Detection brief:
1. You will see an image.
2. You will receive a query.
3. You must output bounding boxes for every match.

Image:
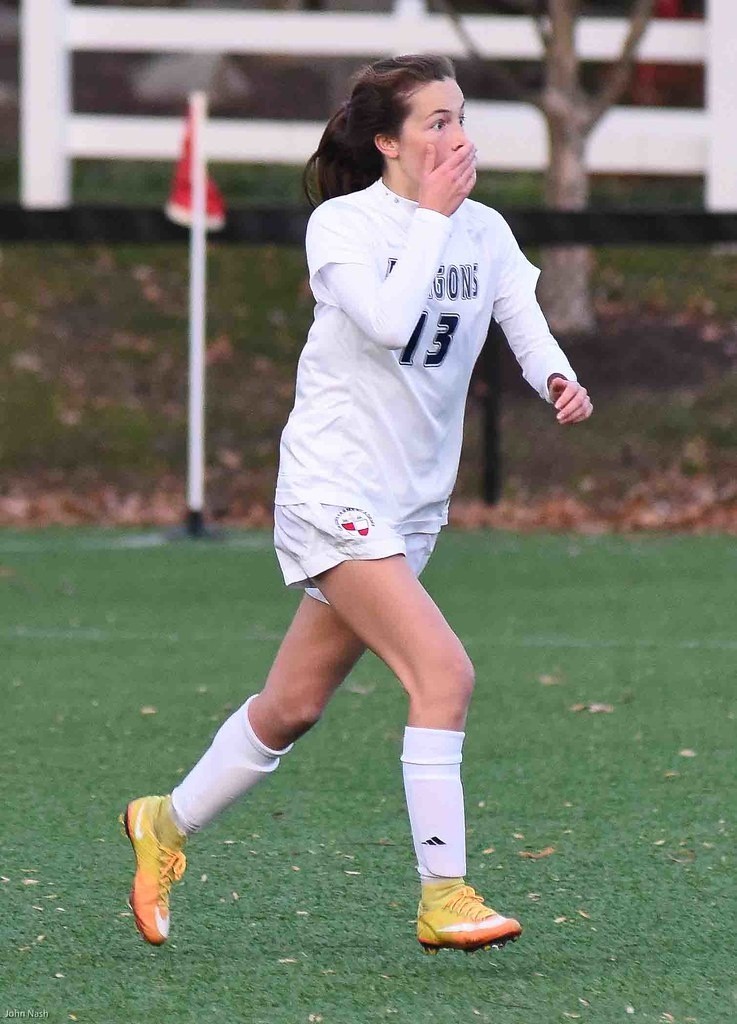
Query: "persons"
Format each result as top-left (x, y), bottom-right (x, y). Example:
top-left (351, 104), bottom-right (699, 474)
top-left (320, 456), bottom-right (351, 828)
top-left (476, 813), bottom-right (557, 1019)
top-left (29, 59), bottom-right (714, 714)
top-left (120, 52), bottom-right (597, 947)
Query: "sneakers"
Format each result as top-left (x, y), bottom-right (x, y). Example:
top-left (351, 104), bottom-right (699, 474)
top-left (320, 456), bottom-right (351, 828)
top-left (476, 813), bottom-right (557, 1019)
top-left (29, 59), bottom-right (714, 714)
top-left (416, 878), bottom-right (522, 957)
top-left (118, 796), bottom-right (186, 946)
